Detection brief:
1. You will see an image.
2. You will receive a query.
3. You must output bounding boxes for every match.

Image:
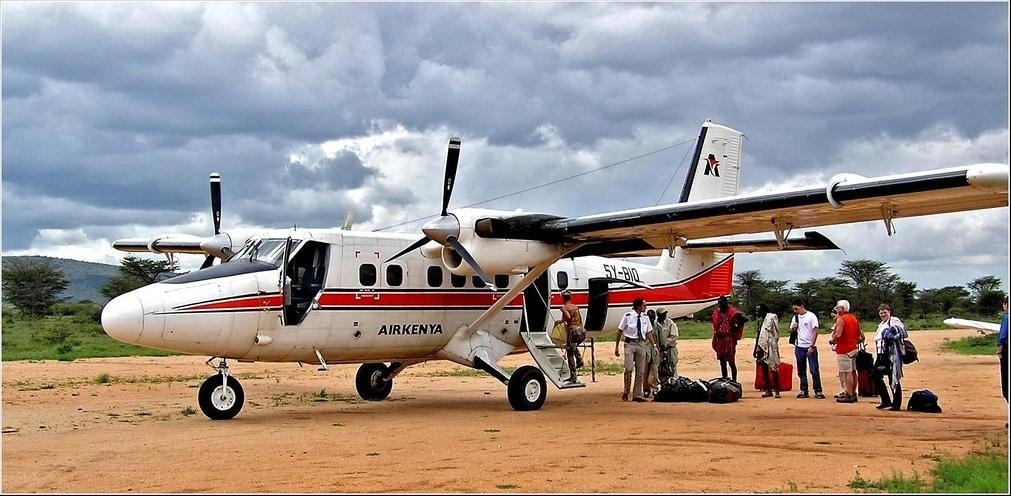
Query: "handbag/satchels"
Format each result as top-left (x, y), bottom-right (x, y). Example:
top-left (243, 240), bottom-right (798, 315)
top-left (901, 340), bottom-right (917, 364)
top-left (908, 390), bottom-right (942, 413)
top-left (657, 359), bottom-right (742, 403)
top-left (789, 329), bottom-right (798, 345)
top-left (857, 352), bottom-right (874, 369)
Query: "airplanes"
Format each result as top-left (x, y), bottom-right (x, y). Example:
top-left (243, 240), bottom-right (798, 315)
top-left (92, 121), bottom-right (1010, 423)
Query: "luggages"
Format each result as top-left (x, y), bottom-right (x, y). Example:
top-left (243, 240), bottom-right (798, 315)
top-left (857, 342), bottom-right (880, 397)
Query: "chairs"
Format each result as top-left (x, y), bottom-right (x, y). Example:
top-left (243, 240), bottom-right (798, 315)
top-left (288, 249), bottom-right (324, 309)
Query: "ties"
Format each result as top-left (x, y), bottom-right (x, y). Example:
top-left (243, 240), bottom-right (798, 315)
top-left (637, 315), bottom-right (644, 340)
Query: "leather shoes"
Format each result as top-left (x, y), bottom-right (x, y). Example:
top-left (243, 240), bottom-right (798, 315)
top-left (876, 402), bottom-right (890, 409)
top-left (887, 407), bottom-right (900, 411)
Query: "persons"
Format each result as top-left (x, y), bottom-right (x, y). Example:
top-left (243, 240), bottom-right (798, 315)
top-left (615, 297), bottom-right (657, 403)
top-left (829, 300), bottom-right (861, 403)
top-left (555, 289), bottom-right (586, 384)
top-left (831, 310), bottom-right (847, 399)
top-left (789, 296), bottom-right (826, 399)
top-left (652, 306), bottom-right (679, 380)
top-left (995, 296), bottom-right (1008, 427)
top-left (712, 296), bottom-right (749, 384)
top-left (753, 305), bottom-right (781, 398)
top-left (872, 304), bottom-right (906, 411)
top-left (643, 309), bottom-right (659, 398)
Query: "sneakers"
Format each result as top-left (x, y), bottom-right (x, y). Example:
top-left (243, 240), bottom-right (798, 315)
top-left (814, 391), bottom-right (825, 399)
top-left (622, 390), bottom-right (658, 402)
top-left (575, 362), bottom-right (583, 368)
top-left (835, 390), bottom-right (857, 403)
top-left (798, 391), bottom-right (809, 398)
top-left (762, 392), bottom-right (781, 398)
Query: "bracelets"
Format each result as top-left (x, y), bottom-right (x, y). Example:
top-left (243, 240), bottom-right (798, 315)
top-left (653, 344), bottom-right (657, 347)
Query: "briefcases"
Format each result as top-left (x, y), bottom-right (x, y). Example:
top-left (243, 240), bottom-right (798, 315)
top-left (755, 361), bottom-right (793, 391)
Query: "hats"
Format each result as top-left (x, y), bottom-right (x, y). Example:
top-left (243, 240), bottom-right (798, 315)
top-left (656, 308), bottom-right (668, 316)
